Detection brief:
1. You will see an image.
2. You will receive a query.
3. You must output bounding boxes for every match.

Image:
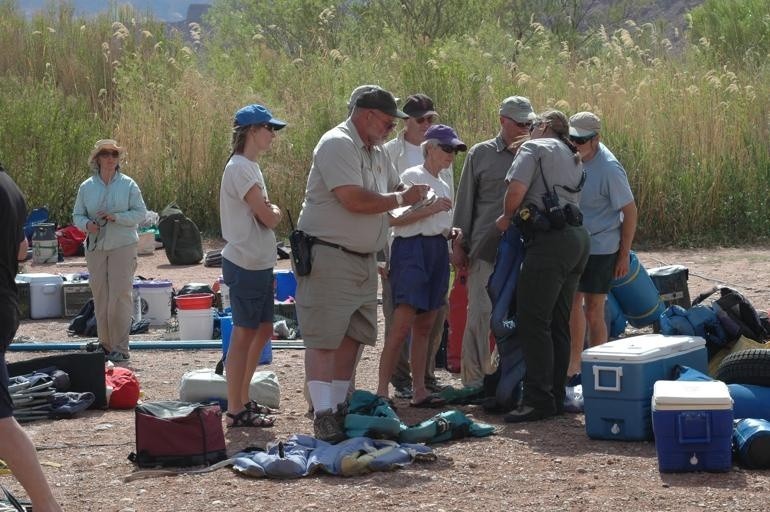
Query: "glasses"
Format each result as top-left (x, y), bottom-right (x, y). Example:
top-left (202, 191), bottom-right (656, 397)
top-left (99, 151), bottom-right (119, 157)
top-left (569, 135), bottom-right (593, 145)
top-left (412, 116), bottom-right (434, 123)
top-left (438, 143), bottom-right (459, 155)
top-left (508, 117), bottom-right (531, 127)
top-left (373, 114), bottom-right (397, 129)
top-left (260, 123), bottom-right (274, 131)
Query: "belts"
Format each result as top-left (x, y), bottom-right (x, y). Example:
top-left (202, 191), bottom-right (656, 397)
top-left (313, 238), bottom-right (368, 258)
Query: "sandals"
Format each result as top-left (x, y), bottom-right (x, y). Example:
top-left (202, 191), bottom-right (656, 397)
top-left (244, 399), bottom-right (277, 414)
top-left (226, 410), bottom-right (276, 427)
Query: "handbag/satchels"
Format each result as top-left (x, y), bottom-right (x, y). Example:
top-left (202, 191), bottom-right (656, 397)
top-left (105, 360), bottom-right (139, 408)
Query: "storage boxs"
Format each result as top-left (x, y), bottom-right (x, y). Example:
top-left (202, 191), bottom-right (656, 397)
top-left (581, 334), bottom-right (708, 443)
top-left (651, 379), bottom-right (732, 474)
top-left (16, 273), bottom-right (63, 318)
top-left (132, 281), bottom-right (172, 326)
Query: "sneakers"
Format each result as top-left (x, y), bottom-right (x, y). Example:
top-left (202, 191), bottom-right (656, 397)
top-left (424, 378), bottom-right (450, 392)
top-left (105, 351), bottom-right (130, 361)
top-left (333, 401), bottom-right (348, 427)
top-left (394, 386), bottom-right (414, 399)
top-left (503, 402), bottom-right (556, 422)
top-left (93, 345), bottom-right (108, 354)
top-left (314, 408), bottom-right (342, 445)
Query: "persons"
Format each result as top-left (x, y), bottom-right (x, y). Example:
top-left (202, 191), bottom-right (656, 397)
top-left (496, 109), bottom-right (592, 423)
top-left (219, 104), bottom-right (285, 427)
top-left (289, 91), bottom-right (410, 446)
top-left (304, 84), bottom-right (401, 415)
top-left (1, 169), bottom-right (63, 511)
top-left (558, 112), bottom-right (638, 387)
top-left (73, 140), bottom-right (146, 362)
top-left (376, 122), bottom-right (468, 408)
top-left (384, 93), bottom-right (456, 400)
top-left (452, 96), bottom-right (541, 392)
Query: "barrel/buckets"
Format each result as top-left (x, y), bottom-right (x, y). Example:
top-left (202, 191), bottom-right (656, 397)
top-left (176, 308), bottom-right (214, 340)
top-left (30, 222), bottom-right (59, 264)
top-left (139, 281), bottom-right (173, 321)
top-left (218, 305), bottom-right (273, 365)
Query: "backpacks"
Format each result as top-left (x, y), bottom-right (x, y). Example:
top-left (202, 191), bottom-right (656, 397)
top-left (158, 203), bottom-right (203, 265)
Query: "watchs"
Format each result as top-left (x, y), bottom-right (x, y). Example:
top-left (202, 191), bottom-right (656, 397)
top-left (394, 192), bottom-right (404, 208)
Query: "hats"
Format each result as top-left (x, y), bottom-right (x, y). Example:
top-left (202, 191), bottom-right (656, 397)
top-left (424, 124), bottom-right (467, 152)
top-left (568, 111), bottom-right (601, 137)
top-left (355, 89), bottom-right (410, 119)
top-left (499, 95), bottom-right (537, 123)
top-left (88, 139), bottom-right (123, 169)
top-left (235, 104), bottom-right (287, 131)
top-left (402, 93), bottom-right (440, 119)
top-left (347, 85), bottom-right (401, 110)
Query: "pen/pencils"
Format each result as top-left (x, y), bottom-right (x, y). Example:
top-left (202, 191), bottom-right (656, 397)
top-left (407, 180), bottom-right (417, 186)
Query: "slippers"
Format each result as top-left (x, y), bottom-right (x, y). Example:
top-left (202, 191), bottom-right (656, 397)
top-left (375, 395), bottom-right (397, 412)
top-left (409, 395), bottom-right (447, 408)
